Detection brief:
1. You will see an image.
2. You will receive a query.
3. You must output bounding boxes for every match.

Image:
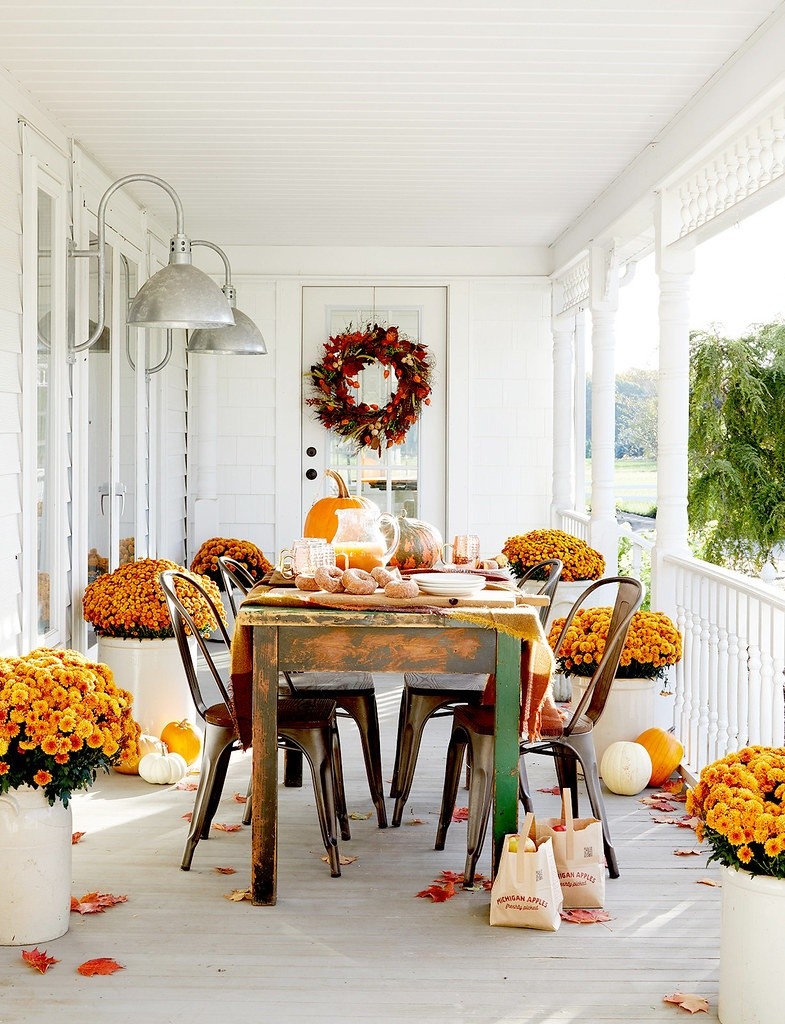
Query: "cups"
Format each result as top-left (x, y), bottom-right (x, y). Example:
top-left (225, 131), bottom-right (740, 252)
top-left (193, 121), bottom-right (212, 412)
top-left (439, 533), bottom-right (480, 566)
top-left (277, 537), bottom-right (349, 580)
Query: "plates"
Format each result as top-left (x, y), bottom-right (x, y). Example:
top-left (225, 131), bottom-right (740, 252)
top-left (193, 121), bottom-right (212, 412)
top-left (411, 572), bottom-right (486, 598)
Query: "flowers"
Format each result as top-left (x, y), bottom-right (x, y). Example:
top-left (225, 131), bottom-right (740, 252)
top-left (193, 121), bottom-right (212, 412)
top-left (0, 647), bottom-right (141, 810)
top-left (33, 535), bottom-right (135, 634)
top-left (81, 556), bottom-right (229, 639)
top-left (545, 604), bottom-right (683, 696)
top-left (302, 315), bottom-right (437, 459)
top-left (190, 537), bottom-right (276, 592)
top-left (686, 743), bottom-right (785, 881)
top-left (501, 528), bottom-right (607, 581)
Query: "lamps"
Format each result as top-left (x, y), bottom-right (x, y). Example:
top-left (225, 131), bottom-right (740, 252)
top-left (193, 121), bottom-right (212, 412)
top-left (66, 174), bottom-right (238, 353)
top-left (118, 239), bottom-right (268, 355)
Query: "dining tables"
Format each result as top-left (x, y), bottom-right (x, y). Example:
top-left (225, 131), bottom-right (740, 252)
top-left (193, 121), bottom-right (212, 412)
top-left (236, 567), bottom-right (542, 909)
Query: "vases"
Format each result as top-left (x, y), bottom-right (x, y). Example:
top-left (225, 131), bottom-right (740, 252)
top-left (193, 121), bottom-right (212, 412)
top-left (516, 577), bottom-right (603, 637)
top-left (198, 587), bottom-right (254, 643)
top-left (0, 778), bottom-right (74, 946)
top-left (569, 673), bottom-right (677, 780)
top-left (717, 862), bottom-right (785, 1023)
top-left (97, 635), bottom-right (198, 771)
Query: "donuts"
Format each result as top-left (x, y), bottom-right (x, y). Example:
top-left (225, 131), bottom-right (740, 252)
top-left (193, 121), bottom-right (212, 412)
top-left (296, 566), bottom-right (418, 599)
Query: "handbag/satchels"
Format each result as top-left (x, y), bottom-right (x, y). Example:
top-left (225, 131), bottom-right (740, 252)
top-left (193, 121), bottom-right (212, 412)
top-left (546, 788), bottom-right (605, 910)
top-left (490, 812), bottom-right (564, 933)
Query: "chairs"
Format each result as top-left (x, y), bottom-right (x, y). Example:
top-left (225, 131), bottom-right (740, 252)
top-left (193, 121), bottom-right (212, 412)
top-left (435, 576), bottom-right (646, 890)
top-left (219, 555), bottom-right (388, 831)
top-left (159, 570), bottom-right (351, 879)
top-left (390, 557), bottom-right (563, 824)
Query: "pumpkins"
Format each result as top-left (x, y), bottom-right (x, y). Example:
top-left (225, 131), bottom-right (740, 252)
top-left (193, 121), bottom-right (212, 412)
top-left (600, 728), bottom-right (684, 796)
top-left (304, 468), bottom-right (443, 571)
top-left (113, 720), bottom-right (205, 785)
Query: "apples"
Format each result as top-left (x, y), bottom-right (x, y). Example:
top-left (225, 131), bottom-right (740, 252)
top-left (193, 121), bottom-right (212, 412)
top-left (508, 825), bottom-right (567, 853)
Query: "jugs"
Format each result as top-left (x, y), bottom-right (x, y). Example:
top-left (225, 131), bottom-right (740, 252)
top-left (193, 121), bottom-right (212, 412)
top-left (331, 508), bottom-right (399, 573)
